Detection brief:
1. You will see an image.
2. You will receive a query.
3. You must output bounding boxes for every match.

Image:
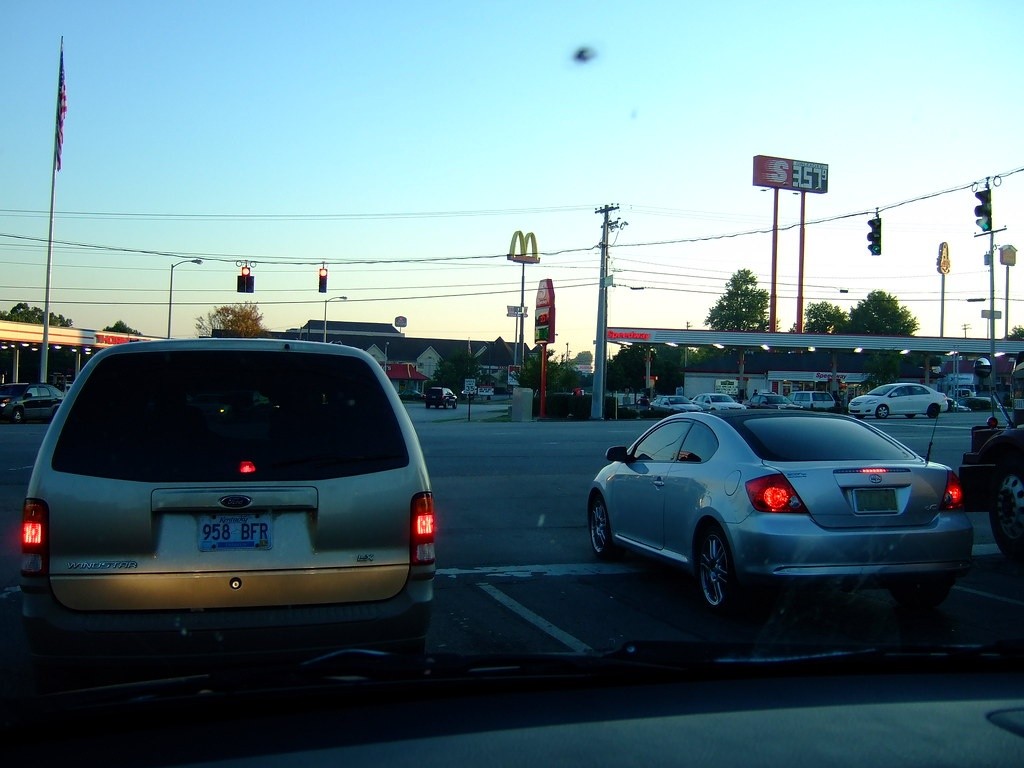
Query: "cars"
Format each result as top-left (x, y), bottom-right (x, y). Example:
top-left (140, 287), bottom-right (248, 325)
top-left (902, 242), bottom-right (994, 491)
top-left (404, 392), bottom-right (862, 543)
top-left (585, 409), bottom-right (975, 615)
top-left (848, 382), bottom-right (949, 419)
top-left (742, 393), bottom-right (804, 410)
top-left (0, 383), bottom-right (66, 423)
top-left (689, 393), bottom-right (747, 410)
top-left (649, 395), bottom-right (704, 411)
top-left (397, 389), bottom-right (426, 400)
top-left (787, 392), bottom-right (836, 412)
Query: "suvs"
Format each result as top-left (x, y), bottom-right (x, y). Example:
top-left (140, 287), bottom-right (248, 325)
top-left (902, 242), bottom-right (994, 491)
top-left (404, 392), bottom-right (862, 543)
top-left (16, 337), bottom-right (438, 666)
top-left (426, 386), bottom-right (458, 409)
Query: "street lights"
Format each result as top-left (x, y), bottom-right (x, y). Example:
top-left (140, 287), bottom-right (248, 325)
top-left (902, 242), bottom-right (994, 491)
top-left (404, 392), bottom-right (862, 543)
top-left (384, 341), bottom-right (389, 373)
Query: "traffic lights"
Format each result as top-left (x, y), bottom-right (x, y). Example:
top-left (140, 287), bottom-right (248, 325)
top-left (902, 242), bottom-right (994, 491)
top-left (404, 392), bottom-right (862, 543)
top-left (975, 190), bottom-right (992, 231)
top-left (319, 269), bottom-right (327, 293)
top-left (867, 218), bottom-right (881, 255)
top-left (238, 267), bottom-right (254, 292)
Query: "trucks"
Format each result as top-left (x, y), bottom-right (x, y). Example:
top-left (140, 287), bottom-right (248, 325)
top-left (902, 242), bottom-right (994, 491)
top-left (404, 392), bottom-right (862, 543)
top-left (958, 351), bottom-right (1024, 560)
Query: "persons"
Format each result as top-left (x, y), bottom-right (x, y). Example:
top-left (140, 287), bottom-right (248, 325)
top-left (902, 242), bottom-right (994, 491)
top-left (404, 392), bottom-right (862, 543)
top-left (636, 396), bottom-right (650, 420)
top-left (753, 389), bottom-right (758, 396)
top-left (573, 386), bottom-right (580, 396)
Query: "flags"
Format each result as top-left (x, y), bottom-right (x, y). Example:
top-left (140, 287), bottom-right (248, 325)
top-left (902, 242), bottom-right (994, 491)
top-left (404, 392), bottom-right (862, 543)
top-left (57, 35), bottom-right (68, 174)
